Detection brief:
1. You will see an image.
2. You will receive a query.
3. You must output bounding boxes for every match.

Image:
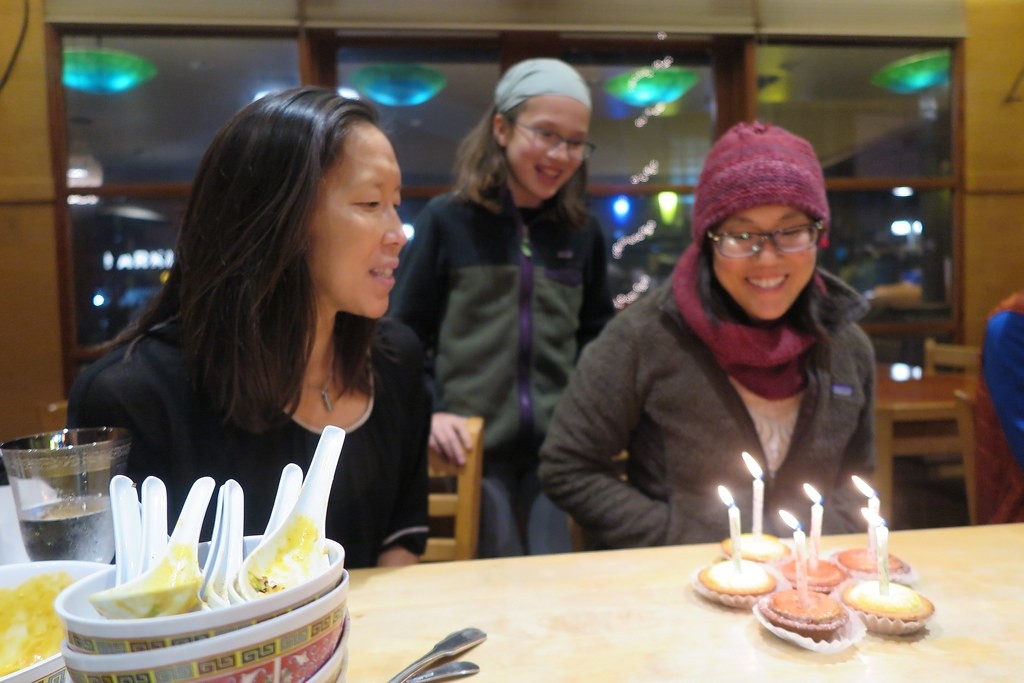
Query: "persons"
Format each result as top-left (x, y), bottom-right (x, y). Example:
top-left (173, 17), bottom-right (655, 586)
top-left (66, 82), bottom-right (434, 571)
top-left (382, 58), bottom-right (618, 559)
top-left (541, 117), bottom-right (877, 552)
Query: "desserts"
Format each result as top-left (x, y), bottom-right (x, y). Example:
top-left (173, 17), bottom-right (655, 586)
top-left (757, 592), bottom-right (849, 641)
top-left (841, 581), bottom-right (934, 623)
top-left (782, 558), bottom-right (849, 593)
top-left (722, 533), bottom-right (791, 564)
top-left (830, 549), bottom-right (910, 576)
top-left (697, 562), bottom-right (776, 597)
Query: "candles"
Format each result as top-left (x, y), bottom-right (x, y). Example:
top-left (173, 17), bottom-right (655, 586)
top-left (719, 452), bottom-right (890, 605)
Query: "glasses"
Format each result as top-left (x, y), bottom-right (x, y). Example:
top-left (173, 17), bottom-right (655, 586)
top-left (516, 120), bottom-right (595, 160)
top-left (707, 218), bottom-right (823, 257)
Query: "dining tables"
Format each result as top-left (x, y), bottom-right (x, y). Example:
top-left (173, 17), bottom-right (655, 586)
top-left (340, 522), bottom-right (1024, 683)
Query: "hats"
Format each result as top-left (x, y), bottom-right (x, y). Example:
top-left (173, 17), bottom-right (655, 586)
top-left (692, 122), bottom-right (828, 242)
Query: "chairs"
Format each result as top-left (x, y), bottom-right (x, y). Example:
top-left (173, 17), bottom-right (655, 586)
top-left (924, 336), bottom-right (980, 380)
top-left (873, 402), bottom-right (976, 526)
top-left (419, 416), bottom-right (484, 562)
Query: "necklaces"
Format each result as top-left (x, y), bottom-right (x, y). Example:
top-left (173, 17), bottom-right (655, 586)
top-left (303, 355), bottom-right (335, 412)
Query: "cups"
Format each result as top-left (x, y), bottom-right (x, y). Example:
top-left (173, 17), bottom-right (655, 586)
top-left (0, 425), bottom-right (131, 566)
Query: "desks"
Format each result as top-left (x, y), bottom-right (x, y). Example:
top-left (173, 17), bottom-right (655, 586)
top-left (878, 363), bottom-right (973, 401)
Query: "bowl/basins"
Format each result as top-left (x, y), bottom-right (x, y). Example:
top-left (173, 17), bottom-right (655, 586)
top-left (0, 559), bottom-right (112, 683)
top-left (66, 609), bottom-right (350, 683)
top-left (336, 649), bottom-right (348, 683)
top-left (62, 570), bottom-right (349, 683)
top-left (55, 537), bottom-right (345, 648)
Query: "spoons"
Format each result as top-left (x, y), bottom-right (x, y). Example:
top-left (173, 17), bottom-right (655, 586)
top-left (87, 425), bottom-right (346, 618)
